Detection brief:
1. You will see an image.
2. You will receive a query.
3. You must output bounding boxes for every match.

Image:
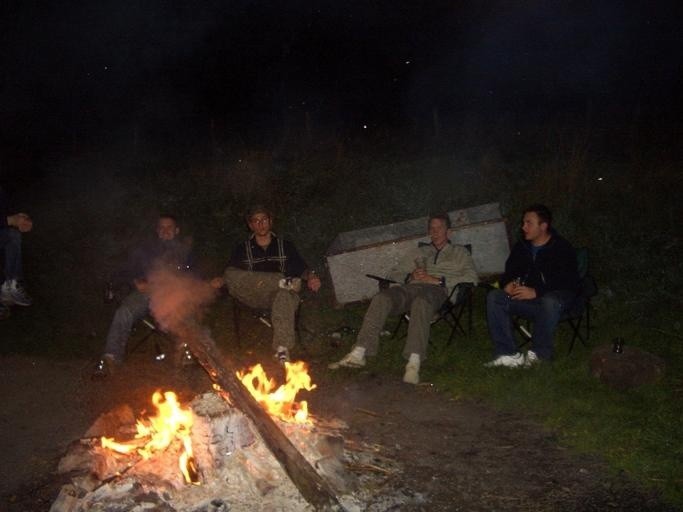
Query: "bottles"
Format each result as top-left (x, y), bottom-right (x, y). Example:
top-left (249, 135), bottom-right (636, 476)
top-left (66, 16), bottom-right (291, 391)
top-left (612, 335), bottom-right (625, 368)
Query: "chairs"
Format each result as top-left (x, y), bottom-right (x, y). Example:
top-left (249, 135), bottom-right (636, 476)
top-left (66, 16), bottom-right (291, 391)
top-left (222, 255), bottom-right (323, 354)
top-left (366, 240), bottom-right (475, 351)
top-left (101, 244), bottom-right (222, 361)
top-left (478, 245), bottom-right (595, 359)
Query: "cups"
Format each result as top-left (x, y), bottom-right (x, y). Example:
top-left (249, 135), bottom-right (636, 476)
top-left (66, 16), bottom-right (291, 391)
top-left (512, 273), bottom-right (528, 290)
top-left (415, 255), bottom-right (427, 273)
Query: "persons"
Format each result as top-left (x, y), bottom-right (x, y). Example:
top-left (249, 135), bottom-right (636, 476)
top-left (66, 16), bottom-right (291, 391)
top-left (479, 202), bottom-right (588, 371)
top-left (207, 203), bottom-right (321, 369)
top-left (326, 209), bottom-right (480, 385)
top-left (0, 212), bottom-right (35, 308)
top-left (89, 214), bottom-right (206, 374)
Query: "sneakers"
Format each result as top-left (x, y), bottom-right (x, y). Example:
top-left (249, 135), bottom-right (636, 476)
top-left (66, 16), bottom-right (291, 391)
top-left (483, 352), bottom-right (526, 370)
top-left (90, 358), bottom-right (109, 380)
top-left (1, 278), bottom-right (34, 307)
top-left (402, 361), bottom-right (420, 386)
top-left (326, 351), bottom-right (368, 370)
top-left (279, 275), bottom-right (302, 292)
top-left (523, 350), bottom-right (539, 371)
top-left (274, 346), bottom-right (289, 363)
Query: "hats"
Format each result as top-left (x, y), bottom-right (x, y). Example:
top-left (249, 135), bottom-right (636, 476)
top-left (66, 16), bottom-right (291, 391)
top-left (245, 205), bottom-right (275, 224)
